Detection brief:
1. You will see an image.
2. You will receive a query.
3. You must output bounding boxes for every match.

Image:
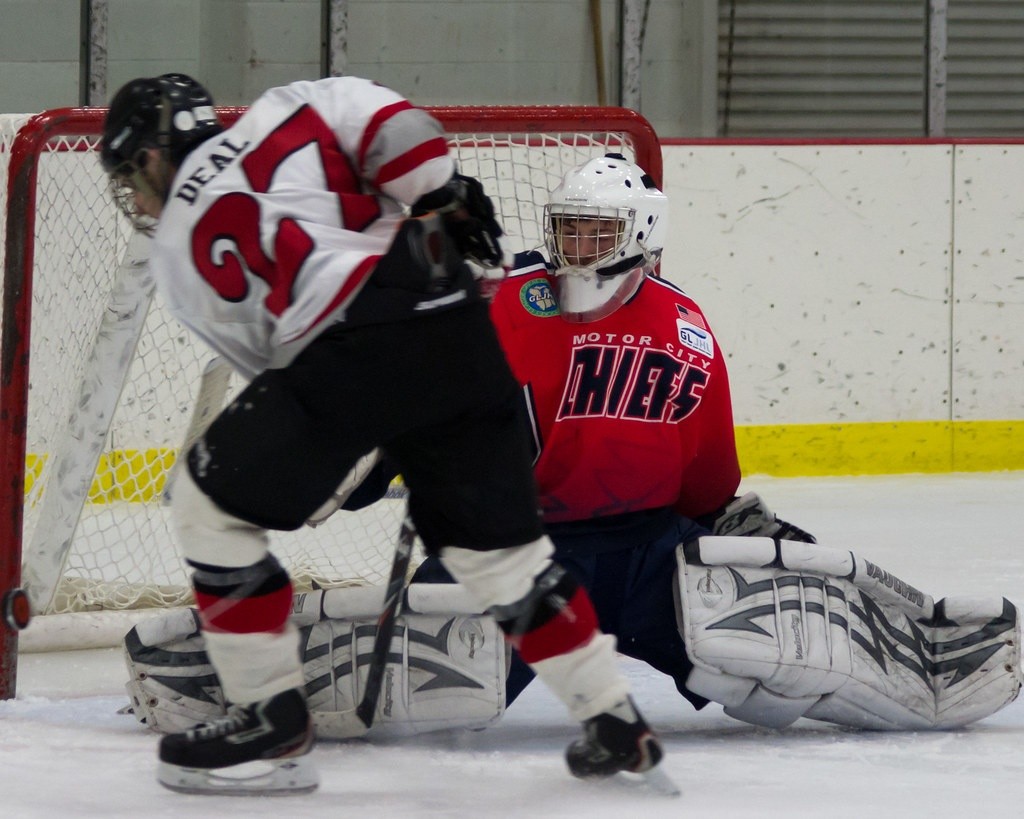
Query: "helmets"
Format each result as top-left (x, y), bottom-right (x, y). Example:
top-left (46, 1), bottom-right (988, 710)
top-left (542, 153), bottom-right (668, 323)
top-left (100, 73), bottom-right (229, 239)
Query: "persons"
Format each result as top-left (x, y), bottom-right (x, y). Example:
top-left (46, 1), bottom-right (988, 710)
top-left (332, 146), bottom-right (1022, 730)
top-left (102, 71), bottom-right (665, 779)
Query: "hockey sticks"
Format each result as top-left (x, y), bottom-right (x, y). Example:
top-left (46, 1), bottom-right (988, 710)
top-left (308, 483), bottom-right (426, 742)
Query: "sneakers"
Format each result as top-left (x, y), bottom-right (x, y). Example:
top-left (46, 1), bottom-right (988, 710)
top-left (564, 692), bottom-right (679, 798)
top-left (157, 686), bottom-right (320, 798)
